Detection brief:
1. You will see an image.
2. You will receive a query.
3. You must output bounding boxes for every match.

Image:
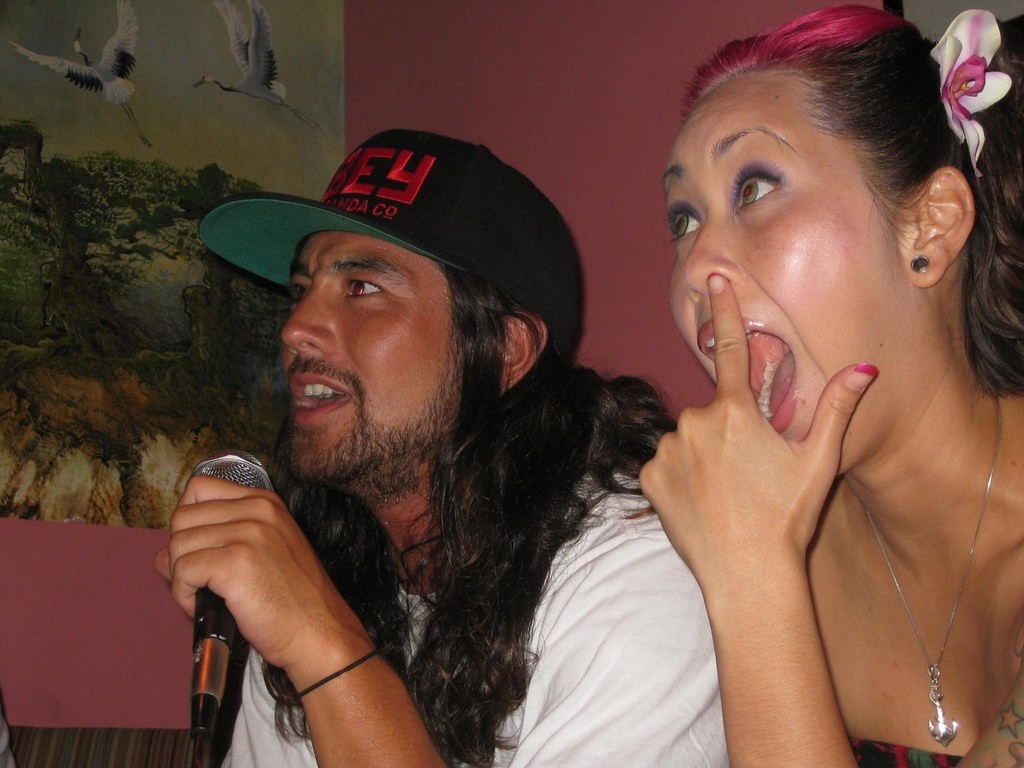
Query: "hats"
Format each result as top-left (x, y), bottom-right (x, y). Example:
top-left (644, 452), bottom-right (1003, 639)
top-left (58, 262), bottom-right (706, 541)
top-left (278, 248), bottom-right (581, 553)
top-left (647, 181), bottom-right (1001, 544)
top-left (195, 129), bottom-right (588, 356)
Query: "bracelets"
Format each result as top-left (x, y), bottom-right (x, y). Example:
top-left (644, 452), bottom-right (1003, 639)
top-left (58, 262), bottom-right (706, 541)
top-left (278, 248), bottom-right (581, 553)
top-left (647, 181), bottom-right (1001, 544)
top-left (296, 650), bottom-right (378, 697)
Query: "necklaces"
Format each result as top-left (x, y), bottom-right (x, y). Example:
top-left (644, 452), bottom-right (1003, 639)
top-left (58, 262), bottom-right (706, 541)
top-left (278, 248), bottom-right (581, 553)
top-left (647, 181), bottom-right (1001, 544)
top-left (861, 395), bottom-right (1005, 749)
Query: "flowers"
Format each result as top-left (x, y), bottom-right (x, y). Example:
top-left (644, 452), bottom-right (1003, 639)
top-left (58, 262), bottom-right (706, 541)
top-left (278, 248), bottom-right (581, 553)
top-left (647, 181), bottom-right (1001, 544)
top-left (928, 9), bottom-right (1014, 179)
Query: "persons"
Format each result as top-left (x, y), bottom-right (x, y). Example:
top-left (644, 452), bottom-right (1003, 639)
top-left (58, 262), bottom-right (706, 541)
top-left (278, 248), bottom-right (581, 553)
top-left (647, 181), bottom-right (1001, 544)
top-left (633, 0), bottom-right (1024, 767)
top-left (148, 125), bottom-right (733, 768)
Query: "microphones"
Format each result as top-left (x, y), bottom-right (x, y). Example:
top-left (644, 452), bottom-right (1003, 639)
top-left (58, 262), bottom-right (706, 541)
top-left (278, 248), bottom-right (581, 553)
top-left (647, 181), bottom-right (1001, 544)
top-left (180, 446), bottom-right (279, 747)
